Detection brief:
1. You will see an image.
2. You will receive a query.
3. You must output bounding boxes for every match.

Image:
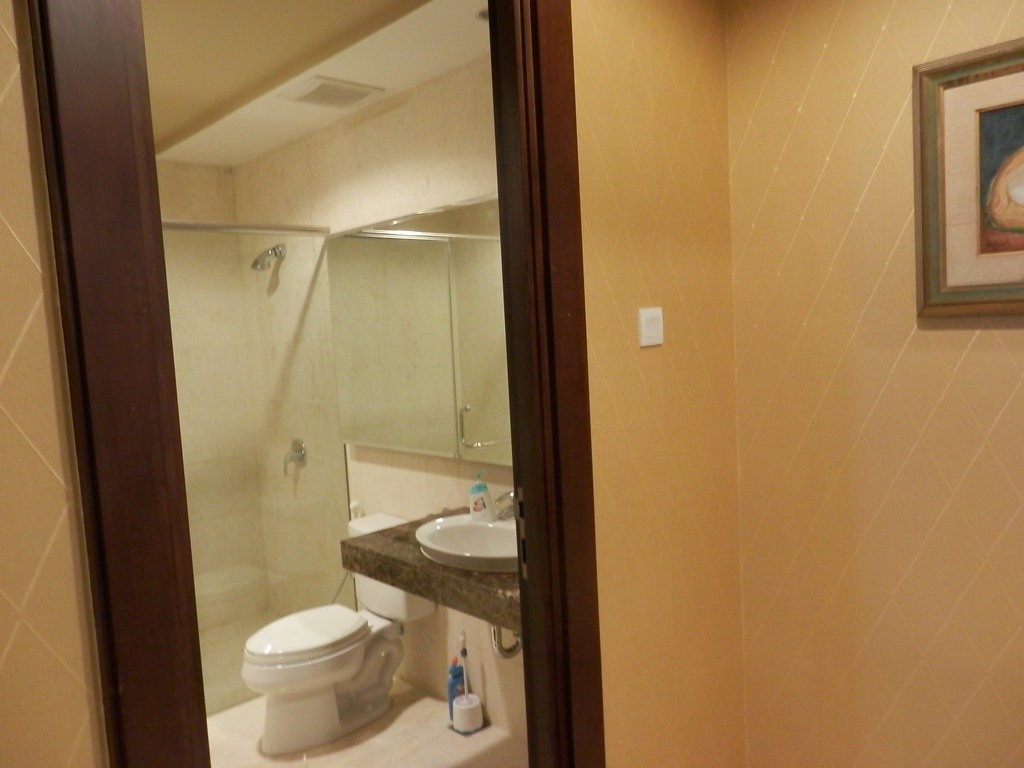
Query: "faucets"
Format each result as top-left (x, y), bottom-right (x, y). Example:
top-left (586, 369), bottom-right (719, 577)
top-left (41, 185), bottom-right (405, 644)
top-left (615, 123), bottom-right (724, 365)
top-left (490, 486), bottom-right (517, 523)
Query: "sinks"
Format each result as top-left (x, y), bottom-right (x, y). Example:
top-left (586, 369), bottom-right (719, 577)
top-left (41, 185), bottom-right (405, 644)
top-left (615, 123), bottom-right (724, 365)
top-left (413, 509), bottom-right (521, 576)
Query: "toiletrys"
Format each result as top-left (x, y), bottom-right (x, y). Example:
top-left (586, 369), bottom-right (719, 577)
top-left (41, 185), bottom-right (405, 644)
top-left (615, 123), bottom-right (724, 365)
top-left (468, 467), bottom-right (492, 519)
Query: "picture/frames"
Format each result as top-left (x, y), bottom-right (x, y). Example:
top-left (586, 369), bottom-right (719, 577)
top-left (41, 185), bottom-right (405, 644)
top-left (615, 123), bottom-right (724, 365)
top-left (912, 34), bottom-right (1023, 321)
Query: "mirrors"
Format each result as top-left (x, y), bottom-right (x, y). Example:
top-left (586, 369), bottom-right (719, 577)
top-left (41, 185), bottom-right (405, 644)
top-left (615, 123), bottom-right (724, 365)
top-left (324, 191), bottom-right (513, 468)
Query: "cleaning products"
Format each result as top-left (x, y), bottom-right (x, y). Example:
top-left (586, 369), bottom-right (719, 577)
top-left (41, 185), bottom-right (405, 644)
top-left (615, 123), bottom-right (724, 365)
top-left (445, 657), bottom-right (472, 724)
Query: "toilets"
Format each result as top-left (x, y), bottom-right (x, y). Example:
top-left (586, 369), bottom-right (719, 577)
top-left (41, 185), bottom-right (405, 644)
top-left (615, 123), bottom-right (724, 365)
top-left (237, 508), bottom-right (437, 760)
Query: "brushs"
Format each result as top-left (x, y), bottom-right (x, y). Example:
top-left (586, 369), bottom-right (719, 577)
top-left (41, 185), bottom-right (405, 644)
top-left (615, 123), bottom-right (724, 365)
top-left (452, 628), bottom-right (485, 735)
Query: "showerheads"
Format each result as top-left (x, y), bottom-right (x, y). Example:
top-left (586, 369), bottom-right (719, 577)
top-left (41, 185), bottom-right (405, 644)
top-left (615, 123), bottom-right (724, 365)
top-left (248, 242), bottom-right (288, 272)
